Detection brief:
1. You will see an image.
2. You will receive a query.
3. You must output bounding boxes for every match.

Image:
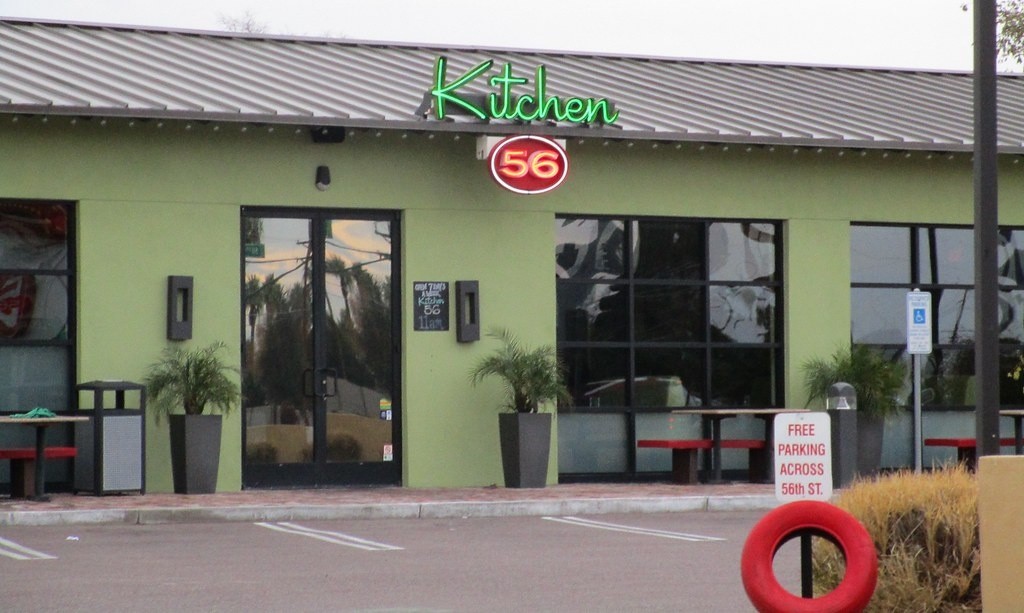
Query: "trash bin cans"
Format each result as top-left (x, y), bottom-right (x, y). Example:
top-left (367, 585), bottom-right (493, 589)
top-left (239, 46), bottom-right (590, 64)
top-left (72, 379), bottom-right (149, 497)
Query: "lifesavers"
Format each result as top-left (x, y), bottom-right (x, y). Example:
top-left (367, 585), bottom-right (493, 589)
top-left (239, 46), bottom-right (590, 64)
top-left (742, 498), bottom-right (877, 613)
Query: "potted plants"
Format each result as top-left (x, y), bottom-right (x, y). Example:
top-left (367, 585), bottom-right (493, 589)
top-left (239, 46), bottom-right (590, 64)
top-left (464, 324), bottom-right (574, 489)
top-left (800, 340), bottom-right (909, 481)
top-left (139, 340), bottom-right (248, 494)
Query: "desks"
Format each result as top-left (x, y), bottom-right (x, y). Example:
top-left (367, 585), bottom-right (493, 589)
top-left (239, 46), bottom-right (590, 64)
top-left (973, 410), bottom-right (1024, 456)
top-left (0, 415), bottom-right (90, 503)
top-left (672, 409), bottom-right (811, 486)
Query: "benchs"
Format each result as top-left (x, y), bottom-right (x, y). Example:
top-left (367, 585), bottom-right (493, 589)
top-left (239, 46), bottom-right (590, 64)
top-left (636, 439), bottom-right (774, 485)
top-left (0, 447), bottom-right (79, 500)
top-left (924, 437), bottom-right (1024, 475)
